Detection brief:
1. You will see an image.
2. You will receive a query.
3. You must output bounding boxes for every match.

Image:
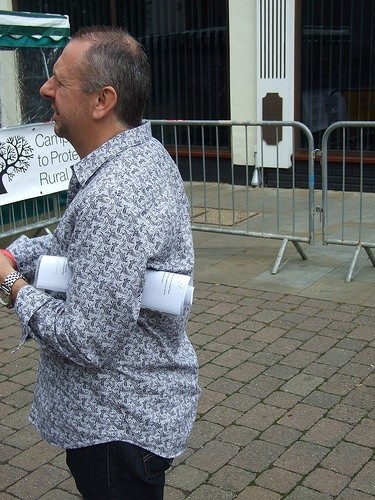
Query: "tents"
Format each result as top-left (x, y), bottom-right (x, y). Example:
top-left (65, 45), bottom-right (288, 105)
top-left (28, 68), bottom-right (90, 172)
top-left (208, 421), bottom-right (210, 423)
top-left (0, 9), bottom-right (71, 80)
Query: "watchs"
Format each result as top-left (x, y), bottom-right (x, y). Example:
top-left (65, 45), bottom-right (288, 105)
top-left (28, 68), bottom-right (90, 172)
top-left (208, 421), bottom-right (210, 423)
top-left (0, 271), bottom-right (26, 309)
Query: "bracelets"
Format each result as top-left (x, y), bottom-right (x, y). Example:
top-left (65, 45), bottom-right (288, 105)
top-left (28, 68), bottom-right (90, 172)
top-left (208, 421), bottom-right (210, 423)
top-left (0, 248), bottom-right (17, 270)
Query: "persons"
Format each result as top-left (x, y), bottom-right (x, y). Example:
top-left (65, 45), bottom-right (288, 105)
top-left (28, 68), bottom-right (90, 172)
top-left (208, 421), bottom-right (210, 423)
top-left (0, 25), bottom-right (201, 500)
